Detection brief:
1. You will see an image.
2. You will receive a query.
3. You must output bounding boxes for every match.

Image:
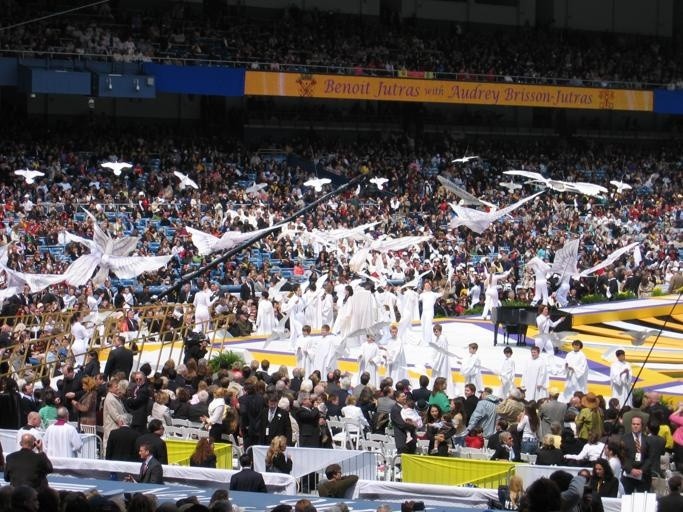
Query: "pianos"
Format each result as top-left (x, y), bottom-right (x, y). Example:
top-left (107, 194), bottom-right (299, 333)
top-left (494, 305), bottom-right (572, 346)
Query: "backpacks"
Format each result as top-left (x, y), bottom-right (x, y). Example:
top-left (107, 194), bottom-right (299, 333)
top-left (371, 412), bottom-right (389, 434)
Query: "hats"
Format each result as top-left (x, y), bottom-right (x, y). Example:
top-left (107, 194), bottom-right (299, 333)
top-left (511, 388), bottom-right (520, 398)
top-left (549, 387), bottom-right (559, 397)
top-left (517, 385), bottom-right (526, 391)
top-left (477, 387), bottom-right (492, 393)
top-left (416, 400), bottom-right (428, 411)
top-left (581, 392), bottom-right (600, 408)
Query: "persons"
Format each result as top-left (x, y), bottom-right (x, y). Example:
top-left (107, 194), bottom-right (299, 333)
top-left (0, 2), bottom-right (682, 511)
